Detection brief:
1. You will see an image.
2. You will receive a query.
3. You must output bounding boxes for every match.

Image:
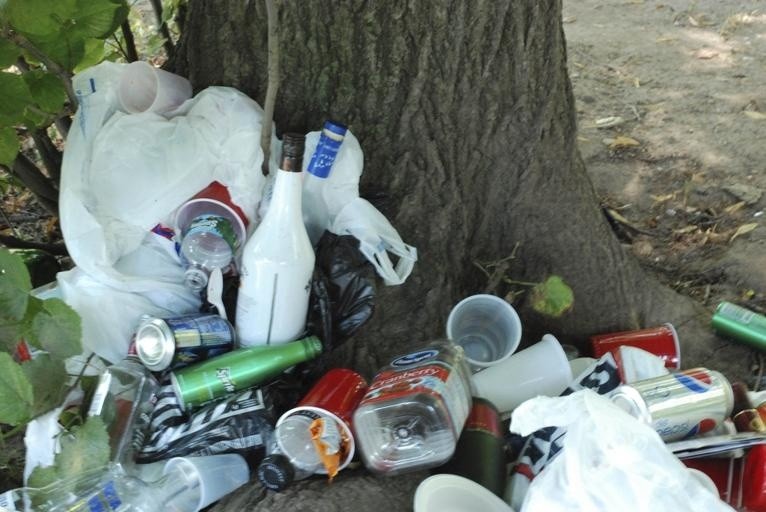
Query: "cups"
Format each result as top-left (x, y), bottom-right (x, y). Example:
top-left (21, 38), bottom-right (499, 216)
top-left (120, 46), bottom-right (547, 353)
top-left (115, 60), bottom-right (193, 116)
top-left (175, 181), bottom-right (248, 252)
top-left (470, 333), bottom-right (574, 416)
top-left (274, 370), bottom-right (368, 476)
top-left (161, 453), bottom-right (249, 512)
top-left (446, 292), bottom-right (521, 371)
top-left (592, 322), bottom-right (682, 383)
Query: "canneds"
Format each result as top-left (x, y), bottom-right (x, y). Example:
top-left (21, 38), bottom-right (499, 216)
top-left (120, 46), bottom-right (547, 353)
top-left (610, 364), bottom-right (735, 443)
top-left (135, 314), bottom-right (234, 374)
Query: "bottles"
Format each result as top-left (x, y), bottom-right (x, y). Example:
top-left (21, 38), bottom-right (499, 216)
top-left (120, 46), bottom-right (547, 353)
top-left (178, 204), bottom-right (241, 294)
top-left (354, 339), bottom-right (473, 476)
top-left (231, 131), bottom-right (315, 348)
top-left (170, 334), bottom-right (323, 408)
top-left (85, 332), bottom-right (160, 473)
top-left (255, 411), bottom-right (328, 492)
top-left (442, 396), bottom-right (507, 497)
top-left (302, 120), bottom-right (351, 248)
